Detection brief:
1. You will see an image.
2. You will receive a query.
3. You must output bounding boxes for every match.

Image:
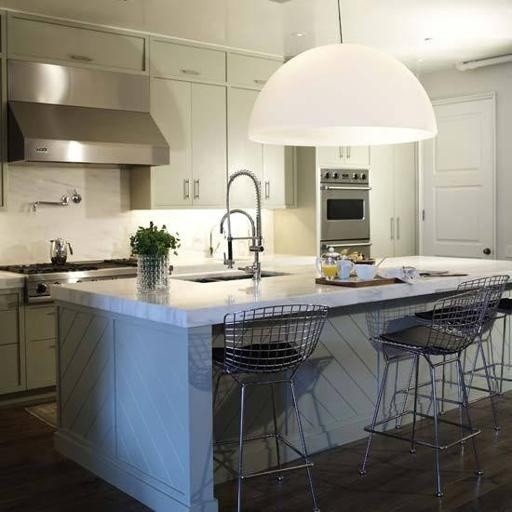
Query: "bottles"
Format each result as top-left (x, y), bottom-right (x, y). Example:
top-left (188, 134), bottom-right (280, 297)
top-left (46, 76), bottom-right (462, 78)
top-left (323, 257), bottom-right (335, 280)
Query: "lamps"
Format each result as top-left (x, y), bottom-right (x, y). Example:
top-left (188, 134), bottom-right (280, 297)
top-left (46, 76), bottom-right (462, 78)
top-left (248, 0), bottom-right (438, 146)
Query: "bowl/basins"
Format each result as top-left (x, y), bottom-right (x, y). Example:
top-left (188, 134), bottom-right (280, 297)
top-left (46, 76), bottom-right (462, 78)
top-left (354, 264), bottom-right (377, 280)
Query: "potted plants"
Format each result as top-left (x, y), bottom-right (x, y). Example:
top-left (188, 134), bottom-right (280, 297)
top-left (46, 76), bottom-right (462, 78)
top-left (129, 221), bottom-right (180, 293)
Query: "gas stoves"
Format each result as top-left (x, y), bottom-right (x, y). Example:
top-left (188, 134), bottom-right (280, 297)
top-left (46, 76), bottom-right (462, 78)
top-left (0, 263), bottom-right (116, 302)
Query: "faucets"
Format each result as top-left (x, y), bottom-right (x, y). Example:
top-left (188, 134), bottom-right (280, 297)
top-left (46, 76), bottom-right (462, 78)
top-left (208, 223), bottom-right (227, 257)
top-left (220, 169), bottom-right (264, 281)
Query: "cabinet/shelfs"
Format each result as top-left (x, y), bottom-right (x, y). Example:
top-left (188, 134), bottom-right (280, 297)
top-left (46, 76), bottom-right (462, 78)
top-left (226, 49), bottom-right (293, 204)
top-left (0, 288), bottom-right (27, 394)
top-left (297, 146), bottom-right (367, 207)
top-left (147, 35), bottom-right (226, 211)
top-left (5, 13), bottom-right (150, 77)
top-left (25, 304), bottom-right (57, 390)
top-left (369, 146), bottom-right (418, 260)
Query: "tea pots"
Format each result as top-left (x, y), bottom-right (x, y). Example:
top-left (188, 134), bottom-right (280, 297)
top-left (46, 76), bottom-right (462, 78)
top-left (316, 247), bottom-right (348, 277)
top-left (48, 238), bottom-right (73, 265)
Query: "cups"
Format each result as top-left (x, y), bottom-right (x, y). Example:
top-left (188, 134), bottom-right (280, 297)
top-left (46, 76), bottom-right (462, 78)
top-left (336, 256), bottom-right (352, 280)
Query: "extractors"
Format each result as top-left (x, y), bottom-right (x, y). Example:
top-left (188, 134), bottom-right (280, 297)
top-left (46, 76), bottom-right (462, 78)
top-left (5, 58), bottom-right (171, 169)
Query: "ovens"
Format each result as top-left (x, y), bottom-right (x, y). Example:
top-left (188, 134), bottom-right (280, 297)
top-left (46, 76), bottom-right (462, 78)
top-left (319, 166), bottom-right (372, 258)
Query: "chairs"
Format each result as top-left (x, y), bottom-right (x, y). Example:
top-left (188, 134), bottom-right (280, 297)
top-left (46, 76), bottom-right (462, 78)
top-left (358, 274), bottom-right (512, 496)
top-left (208, 305), bottom-right (328, 512)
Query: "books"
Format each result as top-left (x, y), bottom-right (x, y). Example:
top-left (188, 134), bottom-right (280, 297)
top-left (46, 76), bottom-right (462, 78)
top-left (414, 257), bottom-right (469, 277)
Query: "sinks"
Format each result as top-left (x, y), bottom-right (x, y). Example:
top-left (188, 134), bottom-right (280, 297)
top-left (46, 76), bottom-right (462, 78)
top-left (169, 270), bottom-right (296, 284)
